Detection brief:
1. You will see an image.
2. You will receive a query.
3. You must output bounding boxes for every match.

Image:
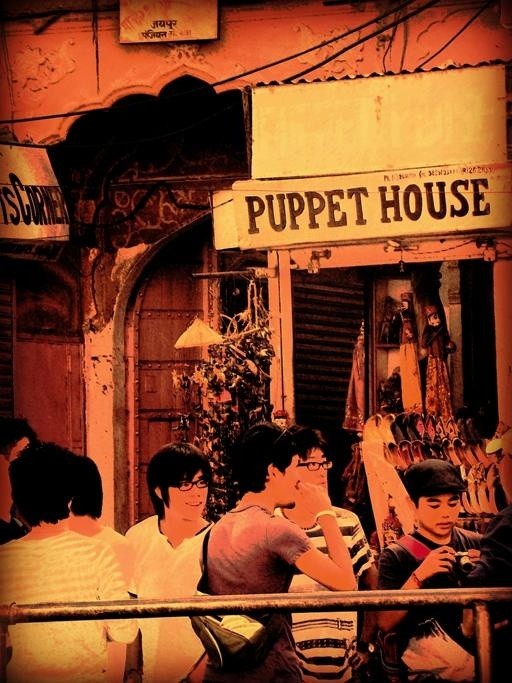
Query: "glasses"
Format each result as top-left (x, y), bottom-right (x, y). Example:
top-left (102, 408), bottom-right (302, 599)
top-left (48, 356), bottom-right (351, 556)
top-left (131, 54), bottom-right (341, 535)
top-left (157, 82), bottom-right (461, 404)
top-left (178, 480), bottom-right (209, 492)
top-left (299, 460), bottom-right (334, 471)
top-left (276, 426), bottom-right (290, 441)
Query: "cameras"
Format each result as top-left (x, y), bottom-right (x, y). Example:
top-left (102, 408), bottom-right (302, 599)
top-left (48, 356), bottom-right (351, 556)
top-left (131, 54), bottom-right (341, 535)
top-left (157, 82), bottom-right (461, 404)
top-left (445, 551), bottom-right (476, 577)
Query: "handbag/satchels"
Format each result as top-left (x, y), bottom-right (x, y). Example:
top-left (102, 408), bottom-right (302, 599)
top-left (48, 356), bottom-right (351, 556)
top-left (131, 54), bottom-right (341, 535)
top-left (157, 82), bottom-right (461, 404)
top-left (375, 629), bottom-right (400, 682)
top-left (401, 617), bottom-right (475, 682)
top-left (190, 590), bottom-right (269, 669)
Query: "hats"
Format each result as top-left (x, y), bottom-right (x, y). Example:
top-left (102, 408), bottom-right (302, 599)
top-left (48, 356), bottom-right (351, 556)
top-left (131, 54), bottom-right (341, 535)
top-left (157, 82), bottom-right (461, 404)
top-left (485, 428), bottom-right (512, 455)
top-left (405, 459), bottom-right (468, 496)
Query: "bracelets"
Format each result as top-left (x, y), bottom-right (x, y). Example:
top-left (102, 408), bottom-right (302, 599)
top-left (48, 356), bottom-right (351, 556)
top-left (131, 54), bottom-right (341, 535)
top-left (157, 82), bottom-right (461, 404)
top-left (315, 510), bottom-right (336, 522)
top-left (411, 572), bottom-right (422, 586)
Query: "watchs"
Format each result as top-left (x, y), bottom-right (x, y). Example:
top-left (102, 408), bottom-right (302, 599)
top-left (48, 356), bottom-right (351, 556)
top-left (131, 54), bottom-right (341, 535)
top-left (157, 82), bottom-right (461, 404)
top-left (358, 640), bottom-right (375, 653)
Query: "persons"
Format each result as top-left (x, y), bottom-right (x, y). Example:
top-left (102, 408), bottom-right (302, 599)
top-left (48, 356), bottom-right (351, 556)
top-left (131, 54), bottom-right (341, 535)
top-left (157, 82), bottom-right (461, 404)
top-left (375, 460), bottom-right (487, 682)
top-left (272, 423), bottom-right (379, 683)
top-left (199, 420), bottom-right (359, 682)
top-left (125, 444), bottom-right (215, 683)
top-left (462, 430), bottom-right (511, 683)
top-left (0, 420), bottom-right (142, 683)
